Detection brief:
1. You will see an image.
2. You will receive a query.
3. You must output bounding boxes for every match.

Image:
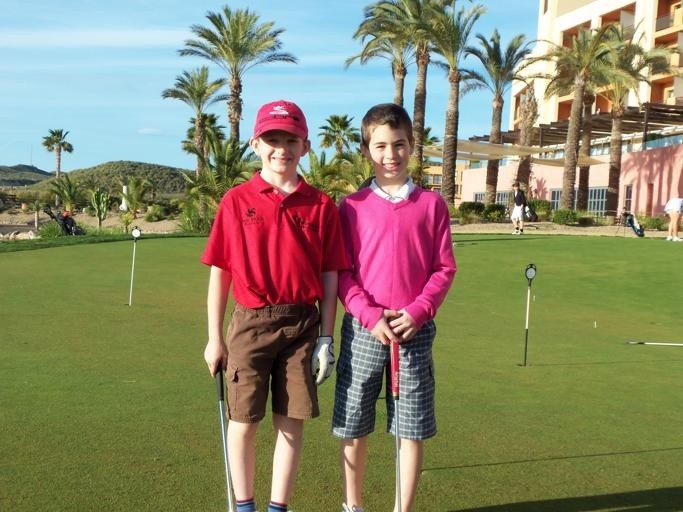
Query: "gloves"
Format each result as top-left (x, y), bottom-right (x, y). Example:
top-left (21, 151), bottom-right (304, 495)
top-left (311, 336), bottom-right (335, 386)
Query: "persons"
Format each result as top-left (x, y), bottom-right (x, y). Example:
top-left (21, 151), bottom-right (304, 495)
top-left (664, 198), bottom-right (683, 241)
top-left (511, 183), bottom-right (530, 235)
top-left (332, 103), bottom-right (456, 512)
top-left (200, 99), bottom-right (353, 512)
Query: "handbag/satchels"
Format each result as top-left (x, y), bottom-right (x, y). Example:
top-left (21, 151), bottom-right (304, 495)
top-left (524, 205), bottom-right (538, 221)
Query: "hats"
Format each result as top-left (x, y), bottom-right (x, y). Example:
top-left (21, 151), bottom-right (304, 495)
top-left (512, 182), bottom-right (520, 187)
top-left (253, 99), bottom-right (308, 140)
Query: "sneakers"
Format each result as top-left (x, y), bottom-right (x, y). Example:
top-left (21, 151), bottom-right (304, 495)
top-left (666, 236), bottom-right (683, 242)
top-left (512, 230), bottom-right (523, 234)
